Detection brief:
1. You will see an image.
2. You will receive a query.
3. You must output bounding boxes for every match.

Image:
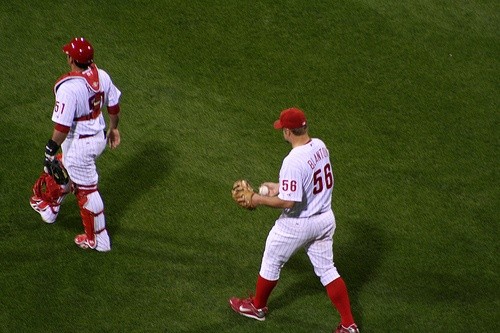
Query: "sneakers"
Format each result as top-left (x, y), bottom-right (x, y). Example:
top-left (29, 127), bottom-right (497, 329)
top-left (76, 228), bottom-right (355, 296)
top-left (229, 297), bottom-right (268, 321)
top-left (29, 195), bottom-right (54, 223)
top-left (76, 234), bottom-right (90, 248)
top-left (334, 322), bottom-right (359, 333)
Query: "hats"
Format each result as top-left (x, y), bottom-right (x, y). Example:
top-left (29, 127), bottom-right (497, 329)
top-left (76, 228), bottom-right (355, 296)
top-left (274, 108), bottom-right (307, 129)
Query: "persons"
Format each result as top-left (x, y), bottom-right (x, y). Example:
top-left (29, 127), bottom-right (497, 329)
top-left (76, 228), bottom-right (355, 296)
top-left (229, 107), bottom-right (360, 333)
top-left (29, 38), bottom-right (121, 252)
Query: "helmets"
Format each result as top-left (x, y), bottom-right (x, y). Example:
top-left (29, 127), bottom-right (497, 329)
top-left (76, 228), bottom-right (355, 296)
top-left (63, 37), bottom-right (93, 62)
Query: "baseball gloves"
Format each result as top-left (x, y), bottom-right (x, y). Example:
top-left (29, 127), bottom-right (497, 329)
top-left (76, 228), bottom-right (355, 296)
top-left (231, 180), bottom-right (257, 210)
top-left (47, 158), bottom-right (70, 186)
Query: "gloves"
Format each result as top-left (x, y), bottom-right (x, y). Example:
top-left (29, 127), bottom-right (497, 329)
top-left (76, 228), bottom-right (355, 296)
top-left (44, 139), bottom-right (59, 174)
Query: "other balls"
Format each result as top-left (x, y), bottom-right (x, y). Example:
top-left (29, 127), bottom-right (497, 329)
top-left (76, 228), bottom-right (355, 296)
top-left (258, 185), bottom-right (269, 196)
